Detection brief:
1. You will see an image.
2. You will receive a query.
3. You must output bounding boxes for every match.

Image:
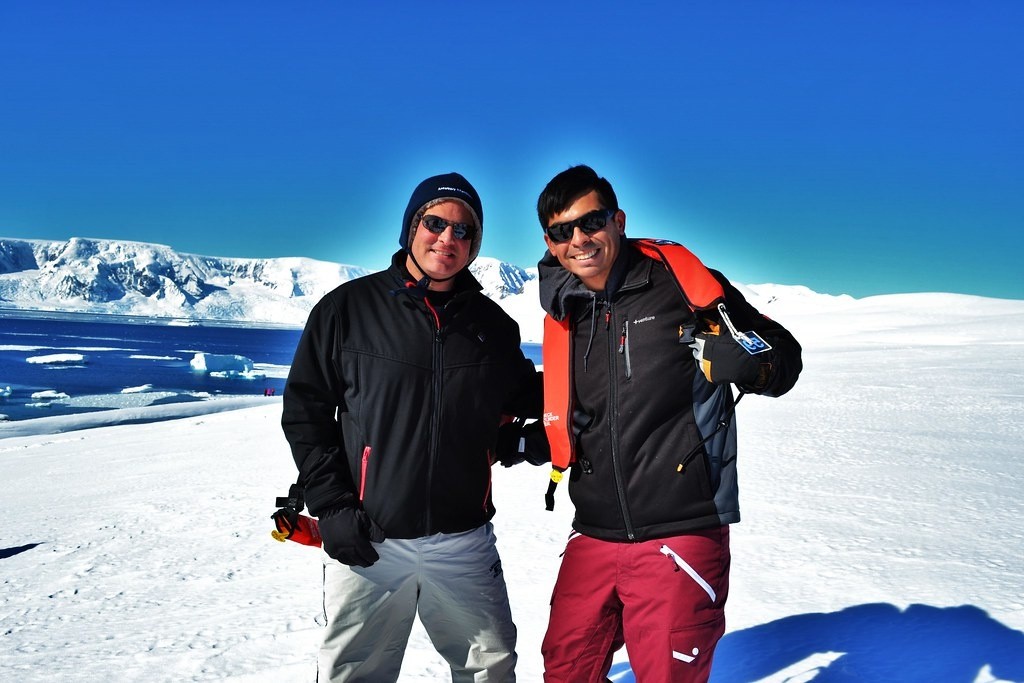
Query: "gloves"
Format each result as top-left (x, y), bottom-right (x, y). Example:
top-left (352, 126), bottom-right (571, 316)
top-left (317, 502), bottom-right (385, 568)
top-left (689, 324), bottom-right (761, 384)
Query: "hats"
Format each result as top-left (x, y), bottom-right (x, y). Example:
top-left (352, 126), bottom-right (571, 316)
top-left (399, 172), bottom-right (484, 268)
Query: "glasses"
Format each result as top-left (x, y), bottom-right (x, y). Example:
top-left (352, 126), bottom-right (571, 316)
top-left (420, 215), bottom-right (475, 241)
top-left (546, 206), bottom-right (612, 243)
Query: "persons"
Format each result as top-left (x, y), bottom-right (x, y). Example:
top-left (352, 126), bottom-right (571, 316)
top-left (499, 164), bottom-right (801, 683)
top-left (280, 170), bottom-right (544, 683)
top-left (264, 388), bottom-right (275, 397)
